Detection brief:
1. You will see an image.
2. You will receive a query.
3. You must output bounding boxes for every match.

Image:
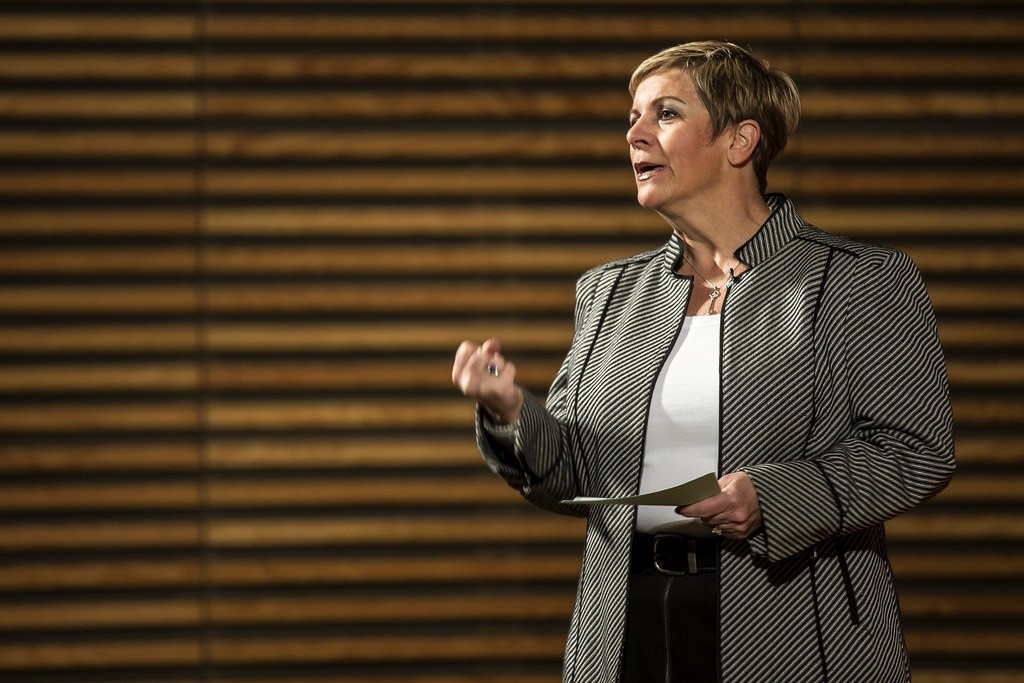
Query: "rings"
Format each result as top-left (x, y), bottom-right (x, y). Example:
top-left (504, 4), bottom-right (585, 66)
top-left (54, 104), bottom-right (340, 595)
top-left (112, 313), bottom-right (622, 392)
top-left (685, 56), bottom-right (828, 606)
top-left (485, 365), bottom-right (502, 378)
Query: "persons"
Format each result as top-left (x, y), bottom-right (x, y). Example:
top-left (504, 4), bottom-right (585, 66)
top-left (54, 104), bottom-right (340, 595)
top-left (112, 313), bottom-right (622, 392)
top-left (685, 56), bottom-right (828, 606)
top-left (451, 43), bottom-right (959, 683)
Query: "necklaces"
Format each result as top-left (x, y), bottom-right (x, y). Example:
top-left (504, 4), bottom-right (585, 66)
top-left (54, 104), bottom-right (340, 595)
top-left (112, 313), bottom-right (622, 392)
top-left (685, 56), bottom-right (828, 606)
top-left (681, 251), bottom-right (742, 315)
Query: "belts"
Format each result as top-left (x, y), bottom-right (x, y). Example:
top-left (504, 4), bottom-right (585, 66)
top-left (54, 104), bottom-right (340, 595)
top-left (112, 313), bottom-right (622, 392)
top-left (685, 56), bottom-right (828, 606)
top-left (629, 532), bottom-right (721, 576)
